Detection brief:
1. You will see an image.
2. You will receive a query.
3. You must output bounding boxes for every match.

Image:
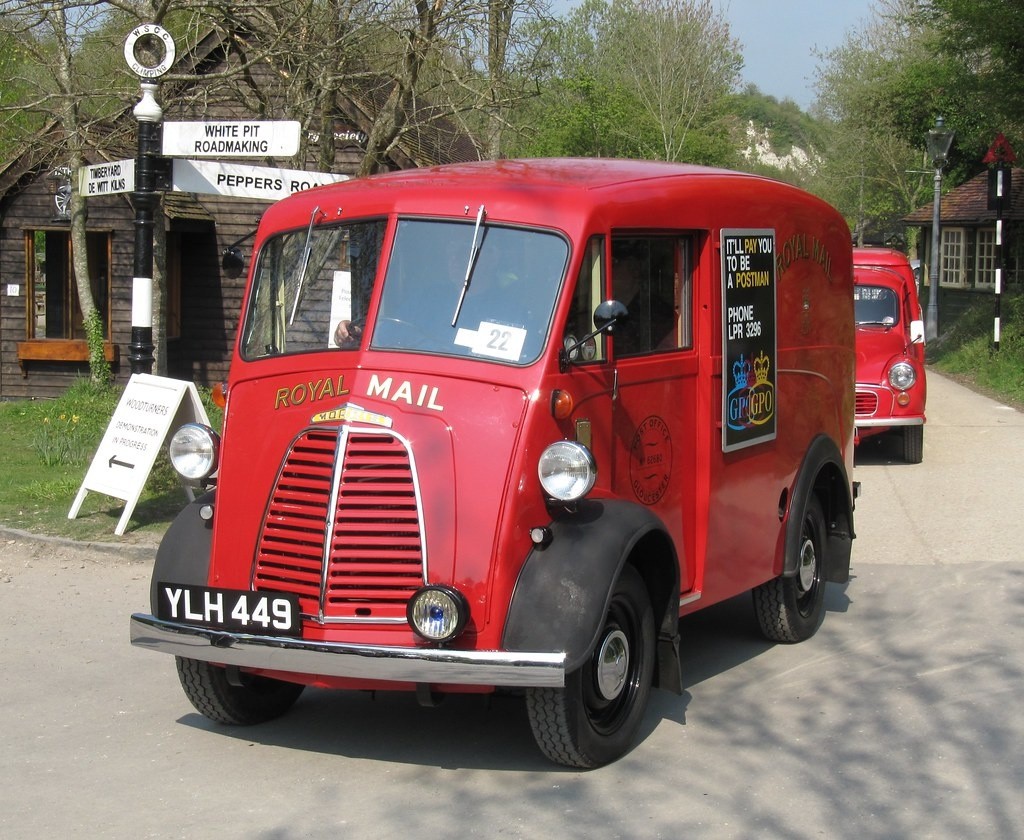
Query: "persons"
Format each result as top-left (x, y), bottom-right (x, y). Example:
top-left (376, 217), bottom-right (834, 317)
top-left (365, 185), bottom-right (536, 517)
top-left (333, 239), bottom-right (498, 347)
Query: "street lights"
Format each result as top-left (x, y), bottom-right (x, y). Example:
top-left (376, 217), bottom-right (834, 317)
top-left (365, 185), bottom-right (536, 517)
top-left (923, 114), bottom-right (957, 357)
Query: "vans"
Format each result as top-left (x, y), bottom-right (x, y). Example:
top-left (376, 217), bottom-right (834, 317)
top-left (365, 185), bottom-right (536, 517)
top-left (852, 248), bottom-right (928, 467)
top-left (129, 157), bottom-right (859, 769)
